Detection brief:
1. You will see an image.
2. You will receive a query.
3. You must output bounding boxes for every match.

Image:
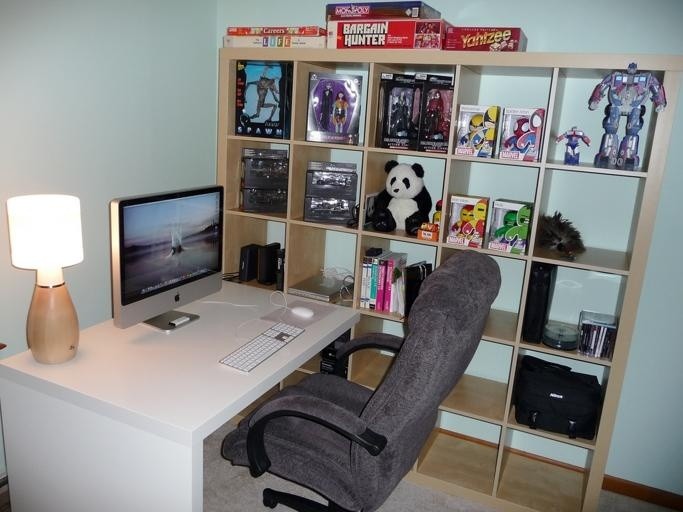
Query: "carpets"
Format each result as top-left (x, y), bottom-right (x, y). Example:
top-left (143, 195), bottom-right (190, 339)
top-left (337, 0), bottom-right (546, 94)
top-left (202, 412), bottom-right (521, 510)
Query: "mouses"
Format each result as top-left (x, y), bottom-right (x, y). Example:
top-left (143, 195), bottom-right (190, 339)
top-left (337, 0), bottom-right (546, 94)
top-left (291, 306), bottom-right (314, 319)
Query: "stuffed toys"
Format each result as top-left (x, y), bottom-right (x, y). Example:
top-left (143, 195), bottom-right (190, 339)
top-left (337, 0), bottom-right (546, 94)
top-left (372, 160), bottom-right (432, 235)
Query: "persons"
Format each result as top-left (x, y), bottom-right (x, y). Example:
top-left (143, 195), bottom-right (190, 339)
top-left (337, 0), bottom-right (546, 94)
top-left (504, 118), bottom-right (530, 153)
top-left (555, 125), bottom-right (591, 163)
top-left (495, 211), bottom-right (517, 246)
top-left (332, 91), bottom-right (348, 134)
top-left (320, 83), bottom-right (333, 129)
top-left (452, 205), bottom-right (475, 240)
top-left (460, 114), bottom-right (483, 150)
top-left (423, 90), bottom-right (450, 141)
top-left (389, 90), bottom-right (408, 138)
top-left (589, 62), bottom-right (666, 169)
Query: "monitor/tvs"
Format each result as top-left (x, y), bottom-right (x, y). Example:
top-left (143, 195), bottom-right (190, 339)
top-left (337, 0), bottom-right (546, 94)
top-left (108, 185), bottom-right (224, 333)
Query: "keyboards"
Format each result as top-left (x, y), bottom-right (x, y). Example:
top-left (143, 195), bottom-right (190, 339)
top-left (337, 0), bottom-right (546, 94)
top-left (218, 322), bottom-right (305, 373)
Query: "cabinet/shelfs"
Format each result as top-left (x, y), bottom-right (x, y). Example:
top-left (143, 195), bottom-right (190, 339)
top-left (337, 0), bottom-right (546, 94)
top-left (213, 35), bottom-right (679, 510)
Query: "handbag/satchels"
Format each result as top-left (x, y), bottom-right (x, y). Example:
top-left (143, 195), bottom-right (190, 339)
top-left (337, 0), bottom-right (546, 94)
top-left (512, 356), bottom-right (603, 441)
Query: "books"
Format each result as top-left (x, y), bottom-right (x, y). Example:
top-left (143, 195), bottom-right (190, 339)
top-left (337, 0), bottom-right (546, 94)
top-left (359, 251), bottom-right (408, 316)
top-left (578, 324), bottom-right (617, 360)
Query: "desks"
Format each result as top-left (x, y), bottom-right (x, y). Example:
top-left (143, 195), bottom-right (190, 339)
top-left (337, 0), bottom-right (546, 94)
top-left (0, 276), bottom-right (364, 512)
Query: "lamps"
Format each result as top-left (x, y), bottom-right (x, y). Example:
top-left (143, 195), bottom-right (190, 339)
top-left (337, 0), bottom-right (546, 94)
top-left (2, 191), bottom-right (91, 368)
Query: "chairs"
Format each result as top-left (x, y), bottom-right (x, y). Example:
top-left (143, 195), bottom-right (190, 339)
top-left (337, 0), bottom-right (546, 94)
top-left (216, 249), bottom-right (505, 512)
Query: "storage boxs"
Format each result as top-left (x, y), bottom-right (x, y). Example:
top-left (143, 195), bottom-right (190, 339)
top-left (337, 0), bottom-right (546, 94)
top-left (324, 17), bottom-right (454, 51)
